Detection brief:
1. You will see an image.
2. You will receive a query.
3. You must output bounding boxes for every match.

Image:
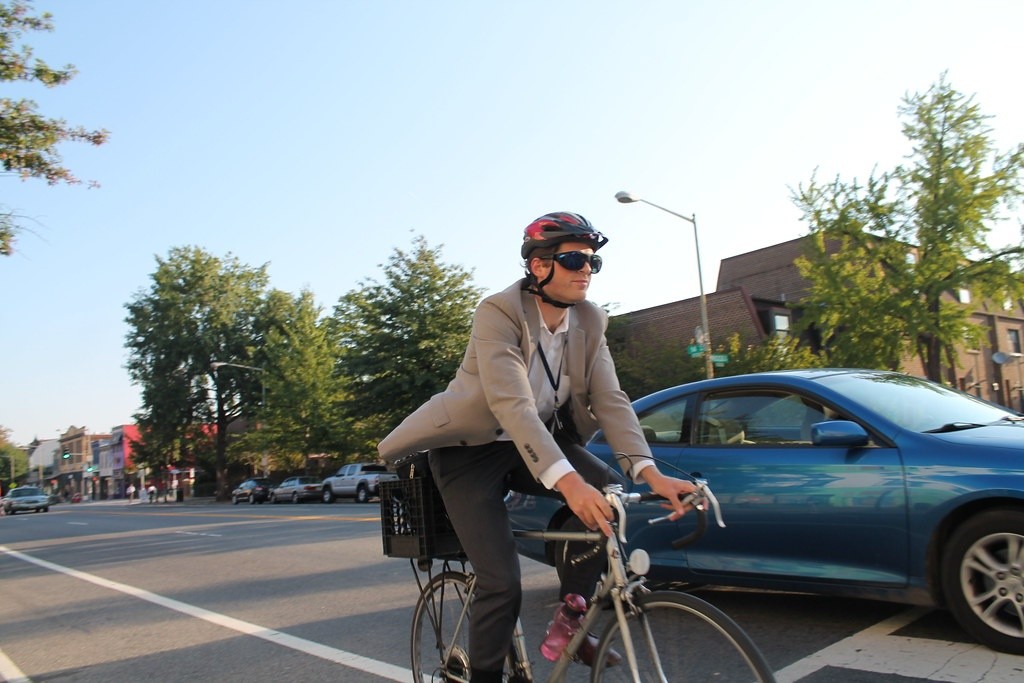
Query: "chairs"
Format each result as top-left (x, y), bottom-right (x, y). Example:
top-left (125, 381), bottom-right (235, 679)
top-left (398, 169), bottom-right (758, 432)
top-left (643, 395), bottom-right (838, 444)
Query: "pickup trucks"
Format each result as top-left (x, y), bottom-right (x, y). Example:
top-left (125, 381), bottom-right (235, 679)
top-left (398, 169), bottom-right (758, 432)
top-left (320, 462), bottom-right (399, 504)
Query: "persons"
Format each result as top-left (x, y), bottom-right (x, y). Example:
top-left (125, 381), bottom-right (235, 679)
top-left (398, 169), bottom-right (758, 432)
top-left (148, 484), bottom-right (156, 504)
top-left (127, 484), bottom-right (136, 503)
top-left (377, 212), bottom-right (708, 683)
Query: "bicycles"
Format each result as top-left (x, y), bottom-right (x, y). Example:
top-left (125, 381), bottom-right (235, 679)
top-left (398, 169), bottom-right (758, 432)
top-left (377, 450), bottom-right (776, 683)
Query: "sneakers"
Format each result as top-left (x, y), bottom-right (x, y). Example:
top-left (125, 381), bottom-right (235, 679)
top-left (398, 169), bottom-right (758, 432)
top-left (541, 594), bottom-right (622, 668)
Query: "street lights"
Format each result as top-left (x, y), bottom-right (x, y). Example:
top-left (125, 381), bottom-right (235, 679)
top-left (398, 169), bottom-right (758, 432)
top-left (210, 362), bottom-right (269, 479)
top-left (614, 189), bottom-right (714, 380)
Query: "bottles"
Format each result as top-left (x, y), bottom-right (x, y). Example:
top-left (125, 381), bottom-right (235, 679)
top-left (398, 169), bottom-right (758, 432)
top-left (540, 593), bottom-right (586, 663)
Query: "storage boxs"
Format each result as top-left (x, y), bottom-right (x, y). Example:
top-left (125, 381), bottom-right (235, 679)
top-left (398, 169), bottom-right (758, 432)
top-left (378, 478), bottom-right (466, 559)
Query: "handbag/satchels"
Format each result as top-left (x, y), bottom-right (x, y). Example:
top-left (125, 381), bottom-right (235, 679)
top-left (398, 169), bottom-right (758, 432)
top-left (394, 450), bottom-right (434, 479)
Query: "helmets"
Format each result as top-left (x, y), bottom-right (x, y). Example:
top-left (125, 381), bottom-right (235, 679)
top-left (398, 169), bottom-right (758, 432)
top-left (521, 211), bottom-right (608, 259)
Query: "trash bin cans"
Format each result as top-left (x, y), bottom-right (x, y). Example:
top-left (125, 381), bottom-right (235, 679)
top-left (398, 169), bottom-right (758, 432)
top-left (176, 488), bottom-right (184, 502)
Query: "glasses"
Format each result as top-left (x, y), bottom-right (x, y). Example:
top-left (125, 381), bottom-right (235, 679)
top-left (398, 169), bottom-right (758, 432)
top-left (539, 251), bottom-right (603, 275)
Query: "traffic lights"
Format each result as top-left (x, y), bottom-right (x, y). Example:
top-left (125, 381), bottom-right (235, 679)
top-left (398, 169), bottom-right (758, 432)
top-left (63, 447), bottom-right (70, 460)
top-left (85, 462), bottom-right (91, 473)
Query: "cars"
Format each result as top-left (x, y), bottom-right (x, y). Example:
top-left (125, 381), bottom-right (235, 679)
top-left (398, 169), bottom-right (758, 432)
top-left (230, 479), bottom-right (280, 506)
top-left (0, 486), bottom-right (51, 515)
top-left (505, 367), bottom-right (1024, 657)
top-left (267, 476), bottom-right (325, 505)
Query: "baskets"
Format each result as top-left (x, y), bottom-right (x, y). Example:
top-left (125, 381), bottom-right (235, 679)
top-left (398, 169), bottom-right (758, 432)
top-left (378, 478), bottom-right (465, 558)
